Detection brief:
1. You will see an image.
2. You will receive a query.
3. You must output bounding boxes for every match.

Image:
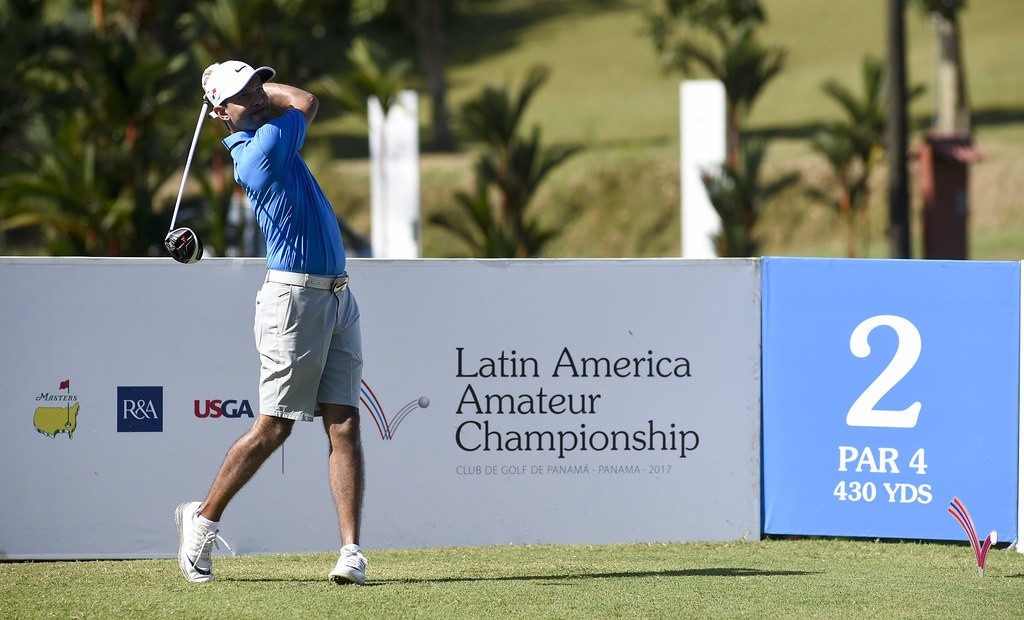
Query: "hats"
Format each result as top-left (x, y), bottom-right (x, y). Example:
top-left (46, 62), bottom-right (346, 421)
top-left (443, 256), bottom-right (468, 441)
top-left (204, 61), bottom-right (277, 107)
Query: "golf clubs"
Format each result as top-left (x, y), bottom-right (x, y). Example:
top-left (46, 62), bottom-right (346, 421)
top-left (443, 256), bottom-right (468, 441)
top-left (163, 100), bottom-right (209, 263)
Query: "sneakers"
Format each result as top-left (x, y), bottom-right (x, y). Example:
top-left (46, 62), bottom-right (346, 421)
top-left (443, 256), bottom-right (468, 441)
top-left (328, 548), bottom-right (369, 585)
top-left (175, 501), bottom-right (234, 582)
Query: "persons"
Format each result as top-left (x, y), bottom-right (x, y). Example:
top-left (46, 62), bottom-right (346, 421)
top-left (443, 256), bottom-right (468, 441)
top-left (174, 60), bottom-right (368, 586)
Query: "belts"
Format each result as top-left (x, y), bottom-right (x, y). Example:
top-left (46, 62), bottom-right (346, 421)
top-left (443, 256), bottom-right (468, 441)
top-left (268, 269), bottom-right (349, 292)
